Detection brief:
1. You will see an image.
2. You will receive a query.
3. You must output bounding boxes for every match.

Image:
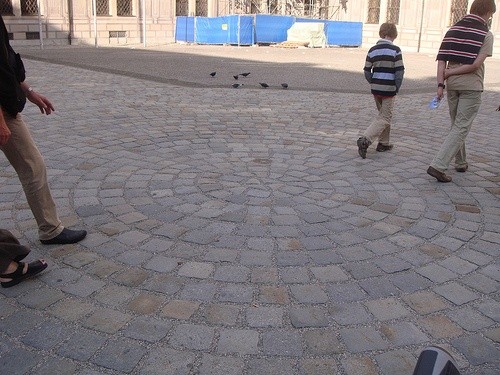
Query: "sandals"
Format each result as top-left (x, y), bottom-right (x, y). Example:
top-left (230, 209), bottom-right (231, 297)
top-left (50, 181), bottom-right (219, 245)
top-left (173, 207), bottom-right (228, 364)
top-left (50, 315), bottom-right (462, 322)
top-left (1, 259), bottom-right (48, 288)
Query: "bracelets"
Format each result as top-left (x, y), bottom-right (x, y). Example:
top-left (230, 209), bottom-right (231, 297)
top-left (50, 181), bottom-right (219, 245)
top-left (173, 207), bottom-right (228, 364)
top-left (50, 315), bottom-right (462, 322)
top-left (24, 86), bottom-right (33, 98)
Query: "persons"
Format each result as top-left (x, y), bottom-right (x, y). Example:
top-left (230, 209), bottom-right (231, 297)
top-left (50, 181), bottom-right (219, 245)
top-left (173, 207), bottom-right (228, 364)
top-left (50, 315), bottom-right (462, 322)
top-left (426, 0), bottom-right (496, 182)
top-left (356, 22), bottom-right (405, 160)
top-left (0, 17), bottom-right (88, 245)
top-left (0, 229), bottom-right (49, 289)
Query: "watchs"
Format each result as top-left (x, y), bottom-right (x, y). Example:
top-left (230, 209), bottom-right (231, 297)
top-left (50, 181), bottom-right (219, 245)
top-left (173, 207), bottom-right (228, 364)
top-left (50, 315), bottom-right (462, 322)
top-left (436, 82), bottom-right (446, 90)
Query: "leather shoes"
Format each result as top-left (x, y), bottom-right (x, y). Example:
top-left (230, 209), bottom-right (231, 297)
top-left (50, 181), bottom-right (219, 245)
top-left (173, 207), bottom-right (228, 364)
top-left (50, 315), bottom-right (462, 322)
top-left (426, 167), bottom-right (453, 182)
top-left (457, 165), bottom-right (468, 173)
top-left (41, 229), bottom-right (87, 245)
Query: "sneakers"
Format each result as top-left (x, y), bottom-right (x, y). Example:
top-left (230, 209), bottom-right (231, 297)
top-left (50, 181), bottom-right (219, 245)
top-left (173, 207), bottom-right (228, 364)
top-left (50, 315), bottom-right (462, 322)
top-left (357, 137), bottom-right (367, 159)
top-left (376, 143), bottom-right (393, 152)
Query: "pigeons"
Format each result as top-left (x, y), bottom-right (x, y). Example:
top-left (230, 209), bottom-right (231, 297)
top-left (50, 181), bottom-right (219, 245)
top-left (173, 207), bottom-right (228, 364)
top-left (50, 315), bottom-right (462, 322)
top-left (239, 72), bottom-right (251, 77)
top-left (209, 72), bottom-right (216, 78)
top-left (259, 82), bottom-right (269, 89)
top-left (232, 83), bottom-right (244, 89)
top-left (280, 83), bottom-right (288, 89)
top-left (233, 74), bottom-right (238, 80)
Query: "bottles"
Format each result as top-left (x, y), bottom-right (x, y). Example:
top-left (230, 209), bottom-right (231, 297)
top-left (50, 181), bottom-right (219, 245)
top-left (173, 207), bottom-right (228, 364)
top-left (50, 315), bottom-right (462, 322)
top-left (428, 96), bottom-right (440, 109)
